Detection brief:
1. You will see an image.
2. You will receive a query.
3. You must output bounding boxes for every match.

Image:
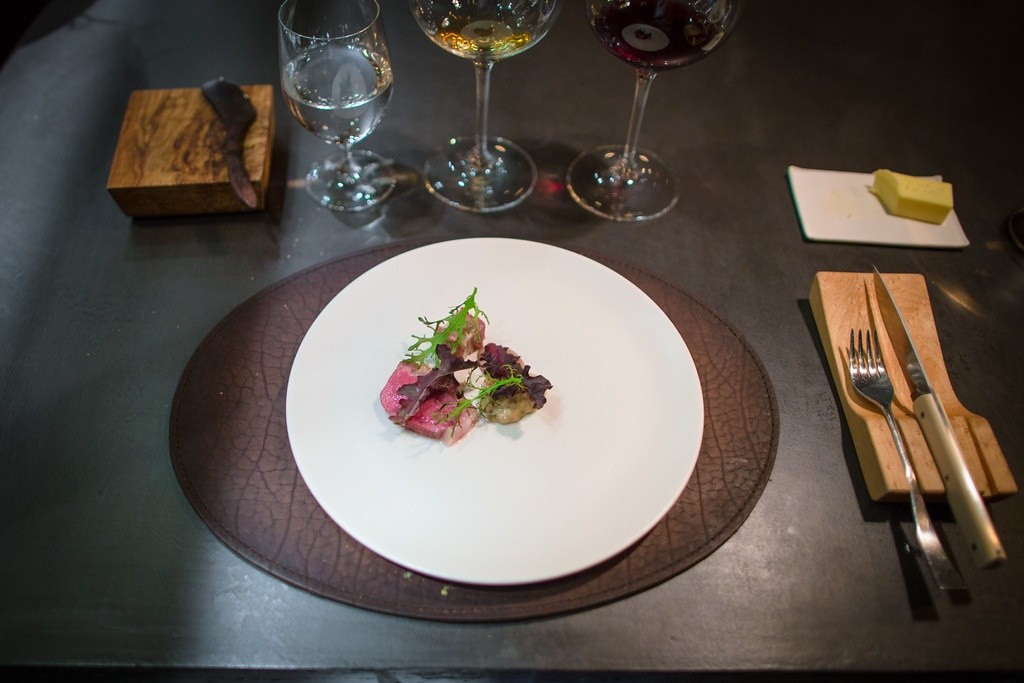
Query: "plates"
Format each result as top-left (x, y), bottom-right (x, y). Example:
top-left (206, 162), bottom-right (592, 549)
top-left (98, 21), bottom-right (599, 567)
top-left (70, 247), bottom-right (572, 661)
top-left (285, 238), bottom-right (704, 586)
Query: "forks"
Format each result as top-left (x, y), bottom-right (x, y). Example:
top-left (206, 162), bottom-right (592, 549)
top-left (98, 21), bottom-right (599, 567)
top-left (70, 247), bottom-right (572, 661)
top-left (850, 328), bottom-right (969, 591)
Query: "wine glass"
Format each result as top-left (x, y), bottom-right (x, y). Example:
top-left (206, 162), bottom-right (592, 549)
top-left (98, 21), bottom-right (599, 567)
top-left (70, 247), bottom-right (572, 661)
top-left (277, 0), bottom-right (395, 213)
top-left (408, 0), bottom-right (564, 213)
top-left (565, 0), bottom-right (744, 221)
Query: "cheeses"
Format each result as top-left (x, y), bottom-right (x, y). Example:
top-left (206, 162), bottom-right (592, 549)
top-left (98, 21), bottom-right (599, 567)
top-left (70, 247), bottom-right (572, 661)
top-left (869, 168), bottom-right (953, 225)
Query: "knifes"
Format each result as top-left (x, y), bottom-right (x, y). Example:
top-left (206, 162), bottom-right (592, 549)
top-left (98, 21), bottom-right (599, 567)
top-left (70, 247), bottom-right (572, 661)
top-left (871, 264), bottom-right (1008, 571)
top-left (202, 77), bottom-right (259, 209)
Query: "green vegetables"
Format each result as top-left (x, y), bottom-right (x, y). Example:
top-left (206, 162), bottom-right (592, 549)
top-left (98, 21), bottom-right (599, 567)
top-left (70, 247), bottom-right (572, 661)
top-left (388, 287), bottom-right (554, 439)
